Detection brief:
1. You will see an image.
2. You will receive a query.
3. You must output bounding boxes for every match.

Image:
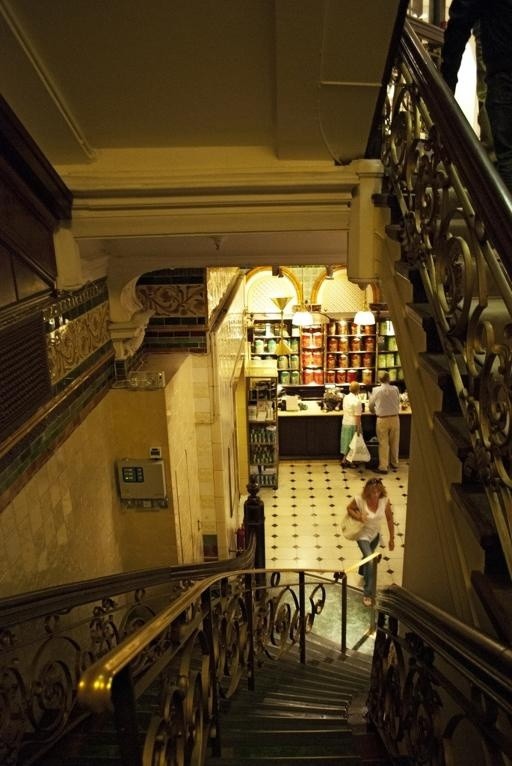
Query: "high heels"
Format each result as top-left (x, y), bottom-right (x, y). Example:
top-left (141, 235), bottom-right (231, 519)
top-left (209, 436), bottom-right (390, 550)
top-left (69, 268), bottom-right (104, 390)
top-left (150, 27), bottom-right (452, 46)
top-left (340, 462), bottom-right (358, 469)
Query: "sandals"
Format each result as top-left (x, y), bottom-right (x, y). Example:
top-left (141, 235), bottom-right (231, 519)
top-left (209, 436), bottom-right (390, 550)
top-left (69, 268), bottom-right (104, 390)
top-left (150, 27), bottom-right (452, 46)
top-left (363, 596), bottom-right (372, 606)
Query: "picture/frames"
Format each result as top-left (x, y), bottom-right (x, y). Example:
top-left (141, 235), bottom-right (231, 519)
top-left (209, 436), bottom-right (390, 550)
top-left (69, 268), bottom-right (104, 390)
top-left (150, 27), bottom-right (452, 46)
top-left (228, 431), bottom-right (236, 518)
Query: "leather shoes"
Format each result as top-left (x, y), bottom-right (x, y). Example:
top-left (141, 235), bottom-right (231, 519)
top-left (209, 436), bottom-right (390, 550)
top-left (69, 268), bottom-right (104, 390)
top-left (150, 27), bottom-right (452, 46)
top-left (393, 466), bottom-right (399, 469)
top-left (373, 469), bottom-right (387, 474)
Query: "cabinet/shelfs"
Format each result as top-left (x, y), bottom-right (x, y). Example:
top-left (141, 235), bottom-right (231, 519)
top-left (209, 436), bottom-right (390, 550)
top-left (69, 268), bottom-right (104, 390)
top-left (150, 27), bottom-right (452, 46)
top-left (246, 359), bottom-right (279, 490)
top-left (248, 312), bottom-right (404, 385)
top-left (285, 383), bottom-right (405, 401)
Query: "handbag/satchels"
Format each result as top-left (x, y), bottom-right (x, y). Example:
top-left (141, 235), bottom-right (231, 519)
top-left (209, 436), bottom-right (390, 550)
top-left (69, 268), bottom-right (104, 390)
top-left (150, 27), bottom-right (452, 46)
top-left (346, 432), bottom-right (370, 462)
top-left (340, 500), bottom-right (367, 540)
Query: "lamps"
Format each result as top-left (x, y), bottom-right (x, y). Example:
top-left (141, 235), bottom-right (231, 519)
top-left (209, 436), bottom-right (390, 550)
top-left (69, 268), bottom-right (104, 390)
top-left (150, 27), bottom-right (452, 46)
top-left (291, 268), bottom-right (313, 327)
top-left (271, 295), bottom-right (292, 356)
top-left (353, 288), bottom-right (376, 325)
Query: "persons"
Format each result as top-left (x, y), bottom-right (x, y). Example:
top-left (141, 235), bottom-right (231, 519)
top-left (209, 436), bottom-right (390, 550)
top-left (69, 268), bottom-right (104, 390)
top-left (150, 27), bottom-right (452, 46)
top-left (368, 369), bottom-right (401, 474)
top-left (338, 381), bottom-right (363, 466)
top-left (438, 0), bottom-right (512, 192)
top-left (471, 21), bottom-right (497, 169)
top-left (346, 477), bottom-right (395, 607)
